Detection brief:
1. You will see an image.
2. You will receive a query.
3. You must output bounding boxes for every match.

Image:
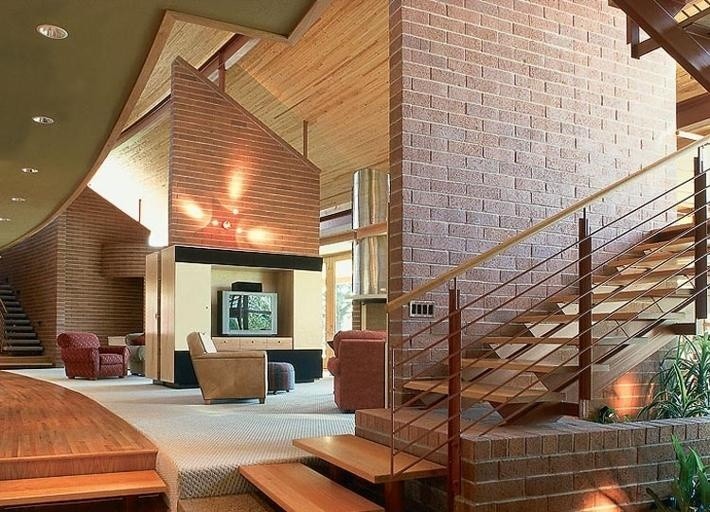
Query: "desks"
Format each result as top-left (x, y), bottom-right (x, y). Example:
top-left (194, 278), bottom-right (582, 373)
top-left (107, 336), bottom-right (128, 347)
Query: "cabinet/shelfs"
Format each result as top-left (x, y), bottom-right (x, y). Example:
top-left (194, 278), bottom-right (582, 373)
top-left (211, 335), bottom-right (292, 353)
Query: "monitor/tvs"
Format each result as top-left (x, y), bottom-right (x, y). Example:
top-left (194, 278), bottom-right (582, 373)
top-left (222, 290), bottom-right (277, 336)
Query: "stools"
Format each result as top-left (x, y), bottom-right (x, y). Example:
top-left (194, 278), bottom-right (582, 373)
top-left (265, 361), bottom-right (296, 394)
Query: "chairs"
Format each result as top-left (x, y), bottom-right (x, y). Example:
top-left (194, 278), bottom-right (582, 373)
top-left (327, 327), bottom-right (388, 413)
top-left (187, 331), bottom-right (270, 404)
top-left (57, 331), bottom-right (129, 380)
top-left (124, 331), bottom-right (145, 378)
